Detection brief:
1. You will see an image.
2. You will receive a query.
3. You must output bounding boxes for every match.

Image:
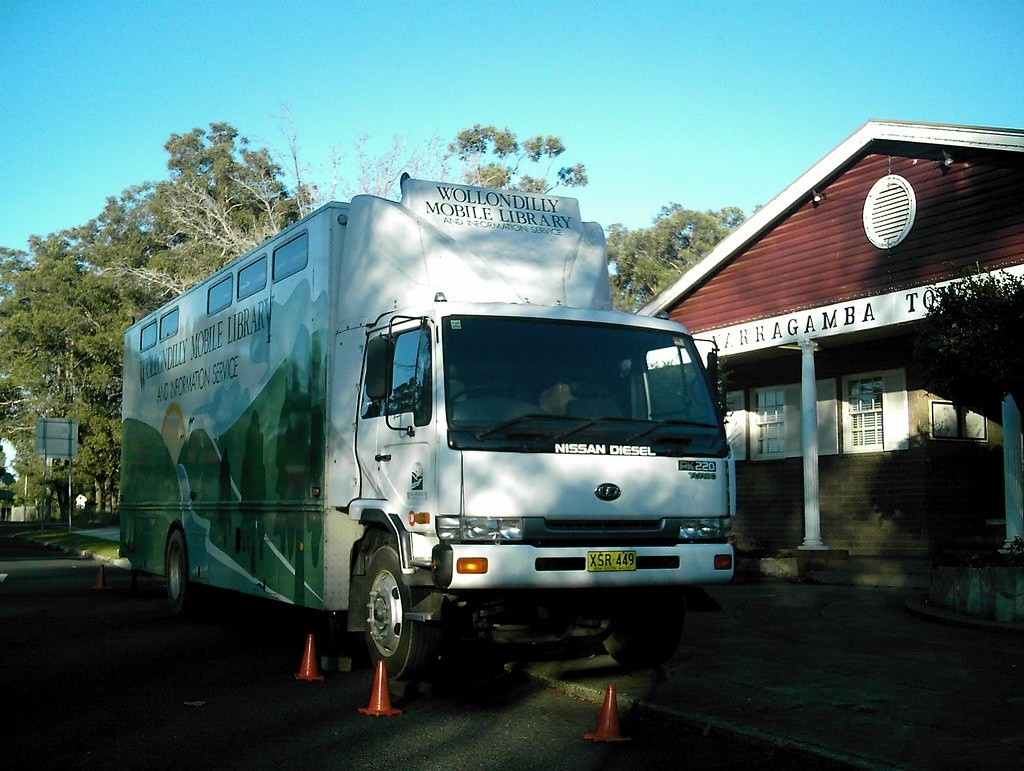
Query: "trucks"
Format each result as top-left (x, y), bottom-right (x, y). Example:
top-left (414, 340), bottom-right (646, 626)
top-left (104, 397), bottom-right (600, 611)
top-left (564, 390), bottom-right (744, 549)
top-left (117, 171), bottom-right (736, 682)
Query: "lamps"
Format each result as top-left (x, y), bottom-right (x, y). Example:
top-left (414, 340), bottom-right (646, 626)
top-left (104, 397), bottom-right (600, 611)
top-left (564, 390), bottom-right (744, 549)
top-left (942, 150), bottom-right (954, 166)
top-left (812, 189), bottom-right (823, 201)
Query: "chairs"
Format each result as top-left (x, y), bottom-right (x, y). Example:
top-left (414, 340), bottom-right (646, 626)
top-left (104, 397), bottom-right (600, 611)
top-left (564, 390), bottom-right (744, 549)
top-left (561, 368), bottom-right (622, 419)
top-left (447, 363), bottom-right (484, 390)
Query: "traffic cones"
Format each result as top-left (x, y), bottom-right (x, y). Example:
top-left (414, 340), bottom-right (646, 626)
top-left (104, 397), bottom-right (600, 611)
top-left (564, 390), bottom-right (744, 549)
top-left (356, 660), bottom-right (404, 717)
top-left (293, 634), bottom-right (324, 681)
top-left (583, 683), bottom-right (632, 744)
top-left (91, 564), bottom-right (115, 591)
